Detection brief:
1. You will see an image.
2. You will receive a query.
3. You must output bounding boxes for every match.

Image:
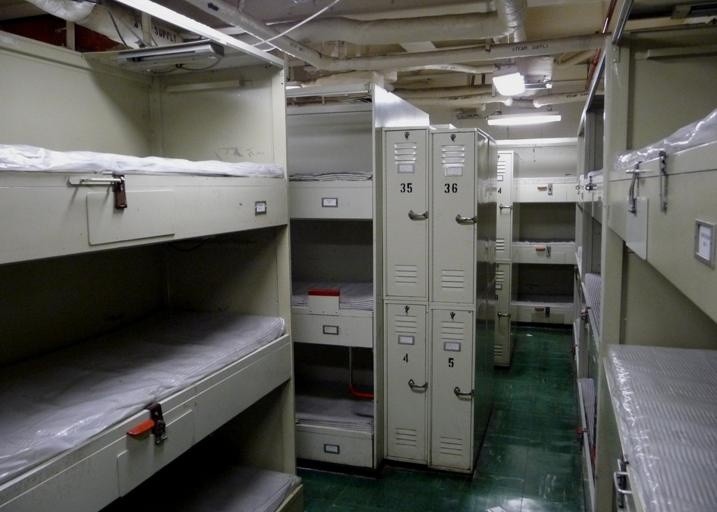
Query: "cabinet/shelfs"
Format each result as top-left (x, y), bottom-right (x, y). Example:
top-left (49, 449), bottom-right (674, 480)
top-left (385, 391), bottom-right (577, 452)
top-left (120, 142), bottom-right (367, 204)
top-left (574, 0), bottom-right (716, 511)
top-left (1, 1), bottom-right (305, 510)
top-left (284, 82), bottom-right (431, 472)
top-left (488, 135), bottom-right (574, 328)
top-left (377, 124), bottom-right (520, 477)
